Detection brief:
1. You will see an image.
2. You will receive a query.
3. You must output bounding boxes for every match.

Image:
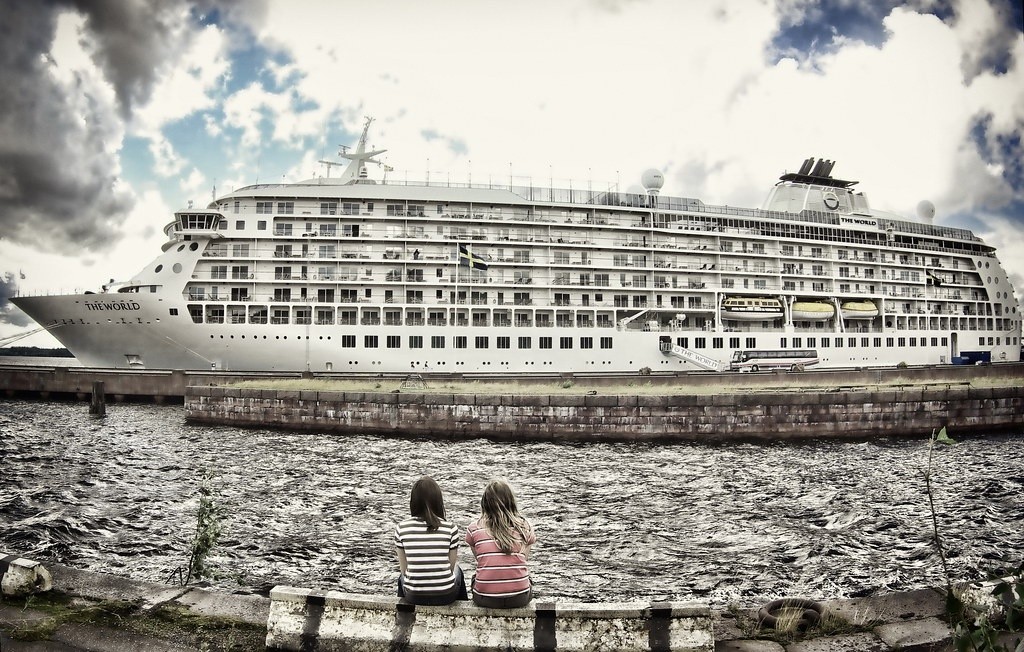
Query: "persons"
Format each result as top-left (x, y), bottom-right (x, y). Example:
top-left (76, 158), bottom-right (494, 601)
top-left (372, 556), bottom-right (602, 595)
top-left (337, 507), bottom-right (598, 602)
top-left (414, 249), bottom-right (419, 259)
top-left (397, 477), bottom-right (468, 605)
top-left (466, 480), bottom-right (534, 609)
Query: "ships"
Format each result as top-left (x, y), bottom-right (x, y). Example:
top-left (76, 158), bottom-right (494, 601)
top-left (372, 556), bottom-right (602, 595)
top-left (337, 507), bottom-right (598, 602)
top-left (9, 117), bottom-right (1021, 374)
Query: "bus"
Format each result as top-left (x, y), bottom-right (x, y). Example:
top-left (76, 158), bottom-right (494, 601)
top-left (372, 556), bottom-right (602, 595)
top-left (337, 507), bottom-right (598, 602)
top-left (731, 349), bottom-right (818, 372)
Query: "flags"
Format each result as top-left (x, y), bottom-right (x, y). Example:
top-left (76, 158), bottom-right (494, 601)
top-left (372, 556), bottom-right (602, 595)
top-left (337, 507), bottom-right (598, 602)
top-left (926, 270), bottom-right (940, 287)
top-left (20, 272), bottom-right (26, 279)
top-left (460, 246), bottom-right (487, 271)
